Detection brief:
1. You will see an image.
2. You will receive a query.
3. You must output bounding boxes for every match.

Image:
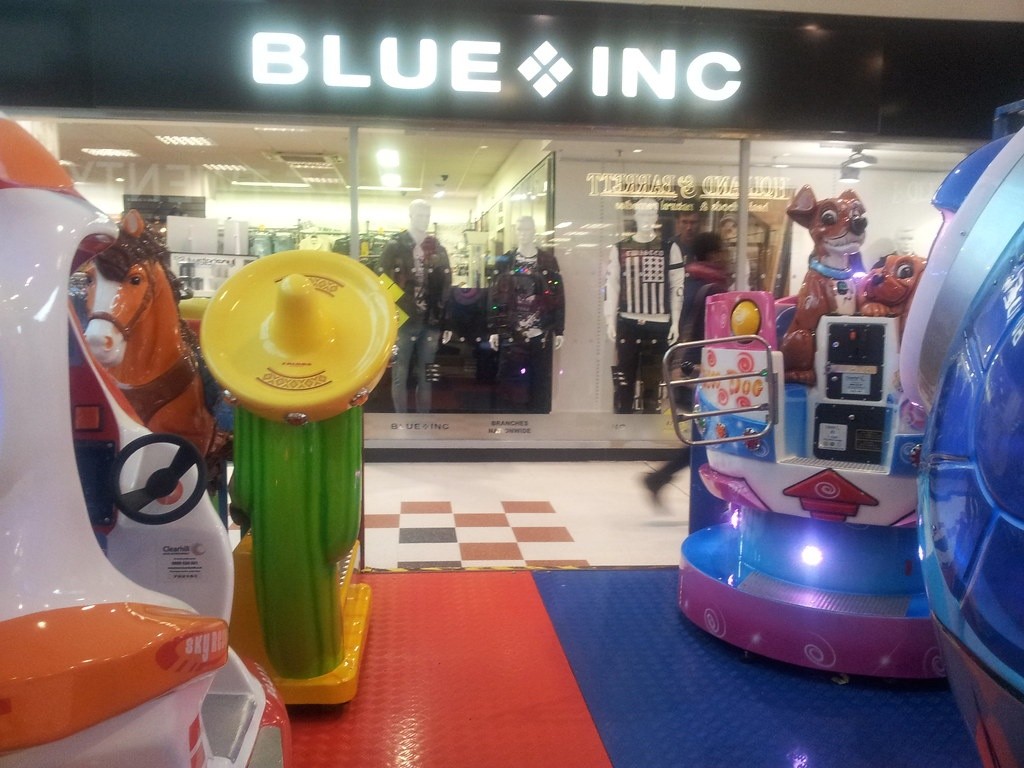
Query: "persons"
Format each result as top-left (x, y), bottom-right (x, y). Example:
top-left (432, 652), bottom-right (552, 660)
top-left (642, 233), bottom-right (732, 496)
top-left (671, 195), bottom-right (702, 276)
top-left (604, 200), bottom-right (685, 414)
top-left (488, 217), bottom-right (566, 412)
top-left (376, 199), bottom-right (452, 413)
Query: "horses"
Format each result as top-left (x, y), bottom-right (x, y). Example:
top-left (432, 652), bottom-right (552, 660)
top-left (77, 211), bottom-right (231, 462)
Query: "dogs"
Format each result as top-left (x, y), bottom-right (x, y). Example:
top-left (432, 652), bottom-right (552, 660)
top-left (780, 184), bottom-right (929, 386)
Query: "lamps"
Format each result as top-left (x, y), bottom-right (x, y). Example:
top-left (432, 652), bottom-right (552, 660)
top-left (841, 154), bottom-right (878, 169)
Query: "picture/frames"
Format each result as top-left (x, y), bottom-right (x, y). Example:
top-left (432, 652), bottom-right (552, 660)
top-left (169, 252), bottom-right (260, 299)
